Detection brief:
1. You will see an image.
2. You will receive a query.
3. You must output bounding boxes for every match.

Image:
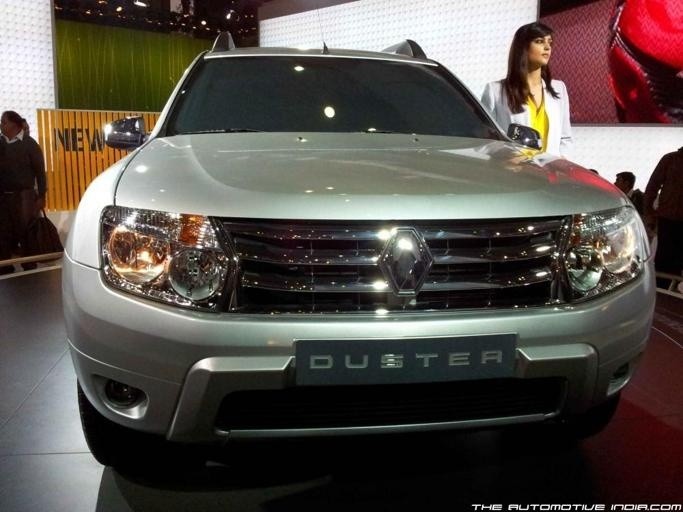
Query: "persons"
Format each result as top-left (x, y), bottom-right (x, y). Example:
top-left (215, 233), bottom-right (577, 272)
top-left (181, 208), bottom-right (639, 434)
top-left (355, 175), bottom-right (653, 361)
top-left (641, 145), bottom-right (683, 288)
top-left (0, 111), bottom-right (47, 274)
top-left (615, 172), bottom-right (653, 243)
top-left (22, 119), bottom-right (29, 135)
top-left (481, 22), bottom-right (575, 162)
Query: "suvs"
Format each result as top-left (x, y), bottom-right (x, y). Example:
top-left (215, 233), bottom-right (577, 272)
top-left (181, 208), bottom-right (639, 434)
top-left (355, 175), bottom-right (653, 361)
top-left (59, 23), bottom-right (658, 478)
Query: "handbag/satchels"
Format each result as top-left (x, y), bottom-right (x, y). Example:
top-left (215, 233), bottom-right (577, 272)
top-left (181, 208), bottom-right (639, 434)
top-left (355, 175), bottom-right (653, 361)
top-left (23, 206), bottom-right (64, 264)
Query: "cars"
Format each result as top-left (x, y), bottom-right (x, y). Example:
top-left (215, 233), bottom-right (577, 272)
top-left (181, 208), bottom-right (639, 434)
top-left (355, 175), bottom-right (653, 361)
top-left (603, 1), bottom-right (682, 126)
